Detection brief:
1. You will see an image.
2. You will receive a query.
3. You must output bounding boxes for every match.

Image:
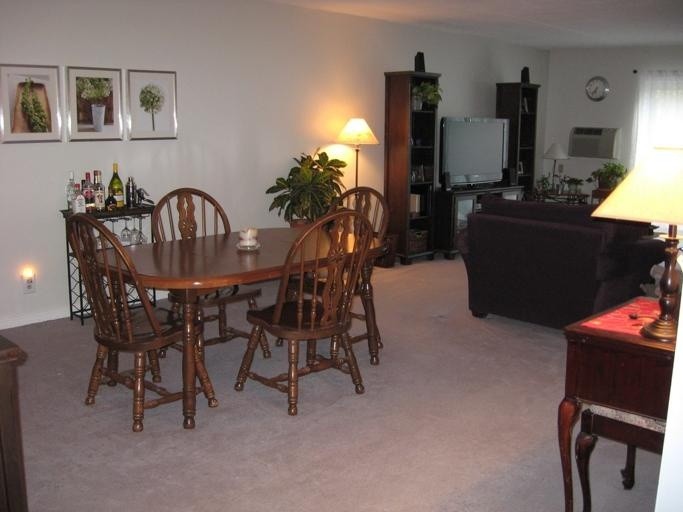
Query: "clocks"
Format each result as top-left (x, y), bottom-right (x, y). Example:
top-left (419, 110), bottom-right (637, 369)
top-left (583, 76), bottom-right (610, 101)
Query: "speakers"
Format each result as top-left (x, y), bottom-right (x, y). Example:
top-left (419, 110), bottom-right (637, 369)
top-left (415, 52), bottom-right (425, 71)
top-left (522, 66), bottom-right (529, 82)
top-left (504, 168), bottom-right (516, 186)
top-left (443, 172), bottom-right (451, 191)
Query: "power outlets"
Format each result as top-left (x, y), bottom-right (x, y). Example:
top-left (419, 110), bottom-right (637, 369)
top-left (22, 275), bottom-right (35, 292)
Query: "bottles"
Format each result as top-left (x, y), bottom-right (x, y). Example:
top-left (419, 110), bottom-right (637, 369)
top-left (71, 184), bottom-right (85, 214)
top-left (125, 176), bottom-right (136, 209)
top-left (92, 171), bottom-right (105, 214)
top-left (104, 187), bottom-right (116, 215)
top-left (79, 180), bottom-right (86, 195)
top-left (65, 172), bottom-right (75, 210)
top-left (81, 173), bottom-right (95, 214)
top-left (108, 162), bottom-right (123, 211)
top-left (92, 171), bottom-right (98, 192)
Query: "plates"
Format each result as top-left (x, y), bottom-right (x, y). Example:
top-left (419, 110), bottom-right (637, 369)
top-left (235, 242), bottom-right (260, 251)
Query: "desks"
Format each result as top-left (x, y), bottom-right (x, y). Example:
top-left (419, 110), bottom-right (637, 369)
top-left (0, 332), bottom-right (30, 512)
top-left (555, 294), bottom-right (682, 512)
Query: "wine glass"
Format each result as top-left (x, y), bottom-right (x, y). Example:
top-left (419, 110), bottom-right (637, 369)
top-left (119, 218), bottom-right (131, 242)
top-left (105, 219), bottom-right (120, 248)
top-left (94, 220), bottom-right (107, 251)
top-left (129, 215), bottom-right (139, 244)
top-left (135, 217), bottom-right (146, 245)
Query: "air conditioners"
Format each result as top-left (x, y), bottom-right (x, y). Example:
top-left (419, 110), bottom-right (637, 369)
top-left (569, 126), bottom-right (622, 160)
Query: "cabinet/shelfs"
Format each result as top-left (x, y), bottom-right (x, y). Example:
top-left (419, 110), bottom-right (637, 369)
top-left (58, 201), bottom-right (159, 328)
top-left (494, 81), bottom-right (541, 199)
top-left (383, 70), bottom-right (442, 265)
top-left (436, 184), bottom-right (524, 260)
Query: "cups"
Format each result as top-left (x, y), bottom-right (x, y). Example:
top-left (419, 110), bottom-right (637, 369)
top-left (90, 104), bottom-right (105, 132)
top-left (239, 224), bottom-right (258, 249)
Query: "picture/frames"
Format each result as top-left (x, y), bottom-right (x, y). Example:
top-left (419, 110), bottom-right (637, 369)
top-left (127, 69), bottom-right (179, 140)
top-left (0, 64), bottom-right (64, 144)
top-left (66, 66), bottom-right (123, 142)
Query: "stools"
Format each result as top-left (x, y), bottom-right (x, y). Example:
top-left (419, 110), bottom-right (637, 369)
top-left (573, 404), bottom-right (666, 512)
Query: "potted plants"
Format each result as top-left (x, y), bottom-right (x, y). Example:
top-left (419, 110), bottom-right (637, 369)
top-left (77, 76), bottom-right (114, 131)
top-left (410, 82), bottom-right (444, 112)
top-left (585, 160), bottom-right (627, 194)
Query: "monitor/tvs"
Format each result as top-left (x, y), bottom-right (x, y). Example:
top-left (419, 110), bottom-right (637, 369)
top-left (440, 116), bottom-right (509, 185)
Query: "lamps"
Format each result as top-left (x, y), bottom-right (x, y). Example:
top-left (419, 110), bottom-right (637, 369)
top-left (336, 117), bottom-right (379, 213)
top-left (590, 146), bottom-right (682, 344)
top-left (542, 142), bottom-right (568, 192)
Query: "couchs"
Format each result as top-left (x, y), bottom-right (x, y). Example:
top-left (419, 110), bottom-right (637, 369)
top-left (453, 197), bottom-right (658, 333)
